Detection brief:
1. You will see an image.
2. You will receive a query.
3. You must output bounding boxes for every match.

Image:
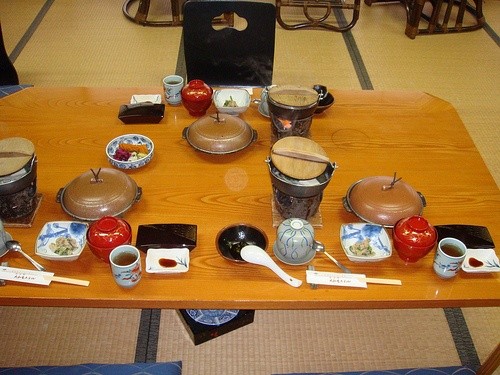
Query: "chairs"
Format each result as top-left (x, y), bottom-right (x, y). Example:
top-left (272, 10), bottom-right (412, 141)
top-left (182, 0), bottom-right (277, 87)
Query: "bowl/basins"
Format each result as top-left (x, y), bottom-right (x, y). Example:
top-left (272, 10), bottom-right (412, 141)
top-left (106, 133), bottom-right (154, 169)
top-left (315, 91), bottom-right (335, 114)
top-left (395, 212), bottom-right (438, 263)
top-left (86, 217), bottom-right (132, 264)
top-left (33, 221), bottom-right (89, 263)
top-left (340, 221), bottom-right (393, 261)
top-left (215, 222), bottom-right (269, 264)
top-left (213, 88), bottom-right (251, 117)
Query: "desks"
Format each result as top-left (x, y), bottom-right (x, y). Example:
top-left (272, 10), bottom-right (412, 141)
top-left (0, 87), bottom-right (500, 375)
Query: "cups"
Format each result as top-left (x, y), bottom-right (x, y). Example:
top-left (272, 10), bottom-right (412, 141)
top-left (109, 245), bottom-right (141, 288)
top-left (276, 216), bottom-right (315, 258)
top-left (433, 237), bottom-right (468, 278)
top-left (163, 74), bottom-right (184, 104)
top-left (260, 84), bottom-right (277, 114)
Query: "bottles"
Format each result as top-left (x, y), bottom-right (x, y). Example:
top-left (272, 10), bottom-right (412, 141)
top-left (181, 79), bottom-right (213, 117)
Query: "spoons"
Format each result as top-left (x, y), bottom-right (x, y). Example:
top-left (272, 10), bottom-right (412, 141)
top-left (4, 240), bottom-right (46, 272)
top-left (312, 240), bottom-right (351, 274)
top-left (241, 243), bottom-right (303, 288)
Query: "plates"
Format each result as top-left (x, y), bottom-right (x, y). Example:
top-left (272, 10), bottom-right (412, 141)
top-left (271, 243), bottom-right (317, 263)
top-left (118, 94), bottom-right (165, 125)
top-left (135, 222), bottom-right (197, 274)
top-left (432, 224), bottom-right (500, 273)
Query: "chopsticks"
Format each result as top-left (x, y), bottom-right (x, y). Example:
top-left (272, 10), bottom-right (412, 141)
top-left (43, 275), bottom-right (90, 287)
top-left (367, 277), bottom-right (401, 285)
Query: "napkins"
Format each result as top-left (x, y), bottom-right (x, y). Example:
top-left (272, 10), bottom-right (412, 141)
top-left (131, 95), bottom-right (162, 104)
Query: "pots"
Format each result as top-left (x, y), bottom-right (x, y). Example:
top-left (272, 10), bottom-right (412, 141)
top-left (1, 153), bottom-right (143, 222)
top-left (182, 110), bottom-right (257, 155)
top-left (263, 88), bottom-right (320, 120)
top-left (265, 156), bottom-right (427, 229)
